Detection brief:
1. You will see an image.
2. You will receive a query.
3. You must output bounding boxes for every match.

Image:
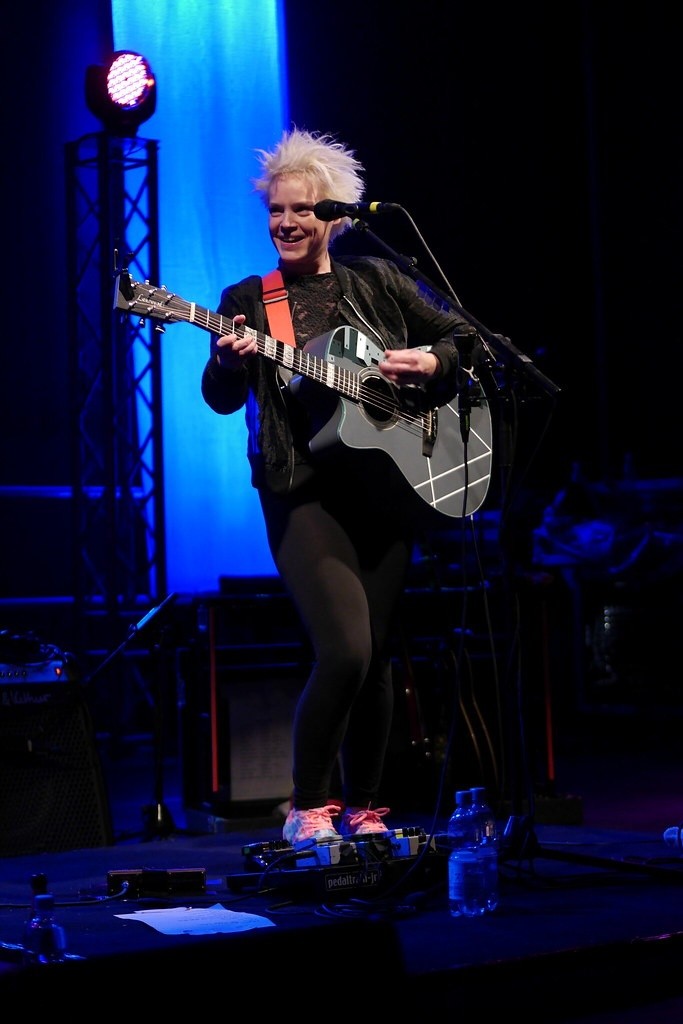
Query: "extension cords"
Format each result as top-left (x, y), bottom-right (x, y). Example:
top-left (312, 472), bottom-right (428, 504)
top-left (106, 868), bottom-right (208, 898)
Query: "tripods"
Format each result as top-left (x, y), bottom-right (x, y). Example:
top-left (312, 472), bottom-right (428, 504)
top-left (357, 218), bottom-right (683, 912)
top-left (25, 591), bottom-right (218, 845)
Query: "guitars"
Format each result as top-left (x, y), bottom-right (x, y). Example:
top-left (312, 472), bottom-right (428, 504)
top-left (112, 264), bottom-right (495, 536)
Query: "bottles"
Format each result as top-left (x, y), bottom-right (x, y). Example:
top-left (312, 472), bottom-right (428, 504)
top-left (25, 895), bottom-right (66, 956)
top-left (467, 787), bottom-right (499, 913)
top-left (447, 789), bottom-right (480, 918)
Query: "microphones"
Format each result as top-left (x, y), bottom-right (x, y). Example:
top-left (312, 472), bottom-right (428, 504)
top-left (314, 198), bottom-right (401, 222)
top-left (0, 741), bottom-right (42, 766)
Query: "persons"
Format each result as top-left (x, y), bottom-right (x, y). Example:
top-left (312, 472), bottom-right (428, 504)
top-left (200, 119), bottom-right (488, 850)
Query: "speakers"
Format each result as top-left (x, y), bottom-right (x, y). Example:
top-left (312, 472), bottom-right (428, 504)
top-left (182, 679), bottom-right (319, 818)
top-left (0, 631), bottom-right (114, 860)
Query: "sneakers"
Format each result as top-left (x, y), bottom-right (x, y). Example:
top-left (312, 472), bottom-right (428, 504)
top-left (345, 807), bottom-right (390, 838)
top-left (282, 805), bottom-right (345, 850)
